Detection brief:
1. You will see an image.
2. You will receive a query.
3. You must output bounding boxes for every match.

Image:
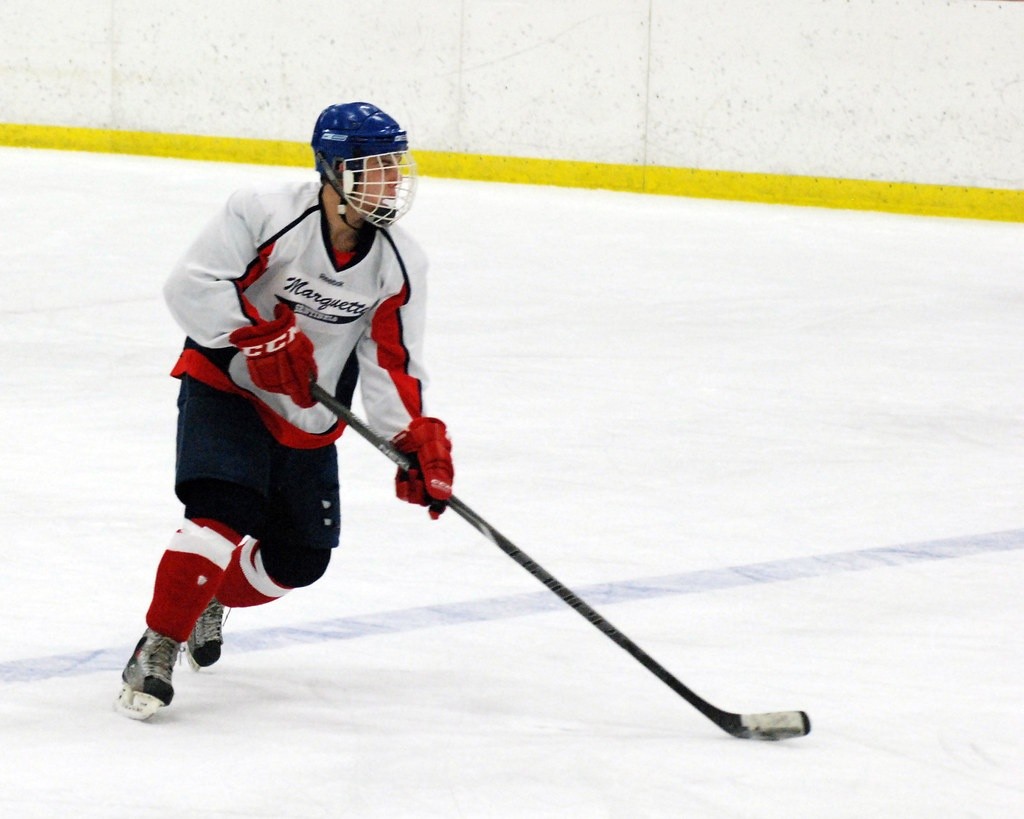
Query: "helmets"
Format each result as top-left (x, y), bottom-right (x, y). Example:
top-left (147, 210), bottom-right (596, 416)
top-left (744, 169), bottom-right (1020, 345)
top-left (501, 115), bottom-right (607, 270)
top-left (312, 101), bottom-right (412, 227)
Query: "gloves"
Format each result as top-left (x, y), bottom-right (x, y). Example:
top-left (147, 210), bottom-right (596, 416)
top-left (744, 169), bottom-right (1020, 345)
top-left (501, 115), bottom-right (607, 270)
top-left (388, 417), bottom-right (453, 520)
top-left (232, 303), bottom-right (318, 409)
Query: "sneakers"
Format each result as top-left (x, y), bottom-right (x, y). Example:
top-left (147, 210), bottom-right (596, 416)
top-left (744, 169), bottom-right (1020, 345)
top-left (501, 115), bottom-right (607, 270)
top-left (184, 593), bottom-right (225, 674)
top-left (118, 625), bottom-right (178, 719)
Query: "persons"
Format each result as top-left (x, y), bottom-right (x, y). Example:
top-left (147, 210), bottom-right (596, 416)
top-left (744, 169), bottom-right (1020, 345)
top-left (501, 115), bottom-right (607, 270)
top-left (114, 103), bottom-right (453, 717)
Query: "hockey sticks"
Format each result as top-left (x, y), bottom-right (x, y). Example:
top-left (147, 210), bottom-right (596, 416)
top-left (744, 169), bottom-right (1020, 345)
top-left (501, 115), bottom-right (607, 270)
top-left (310, 382), bottom-right (814, 742)
